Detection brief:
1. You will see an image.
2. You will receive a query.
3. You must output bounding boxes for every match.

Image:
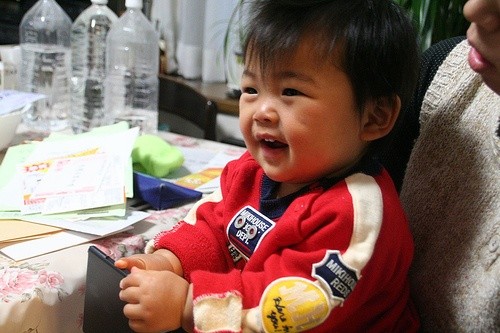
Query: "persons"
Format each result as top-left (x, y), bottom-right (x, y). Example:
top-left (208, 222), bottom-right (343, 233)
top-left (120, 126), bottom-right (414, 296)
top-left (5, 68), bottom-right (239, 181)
top-left (386, 0), bottom-right (500, 333)
top-left (115, 0), bottom-right (415, 333)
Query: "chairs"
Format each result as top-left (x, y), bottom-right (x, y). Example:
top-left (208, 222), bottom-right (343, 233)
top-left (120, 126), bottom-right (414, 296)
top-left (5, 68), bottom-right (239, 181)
top-left (83, 77), bottom-right (218, 141)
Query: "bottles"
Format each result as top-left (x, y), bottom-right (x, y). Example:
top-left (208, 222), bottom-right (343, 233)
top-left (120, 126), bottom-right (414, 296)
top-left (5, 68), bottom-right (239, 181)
top-left (19, 0), bottom-right (73, 130)
top-left (104, 0), bottom-right (159, 136)
top-left (69, 0), bottom-right (119, 133)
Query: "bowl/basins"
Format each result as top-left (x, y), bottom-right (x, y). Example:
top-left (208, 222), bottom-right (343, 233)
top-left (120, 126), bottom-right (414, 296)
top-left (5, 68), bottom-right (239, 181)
top-left (1, 104), bottom-right (33, 152)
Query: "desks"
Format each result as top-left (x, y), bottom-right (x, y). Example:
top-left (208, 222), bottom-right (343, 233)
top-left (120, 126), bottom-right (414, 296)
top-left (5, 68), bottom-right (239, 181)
top-left (0, 123), bottom-right (247, 333)
